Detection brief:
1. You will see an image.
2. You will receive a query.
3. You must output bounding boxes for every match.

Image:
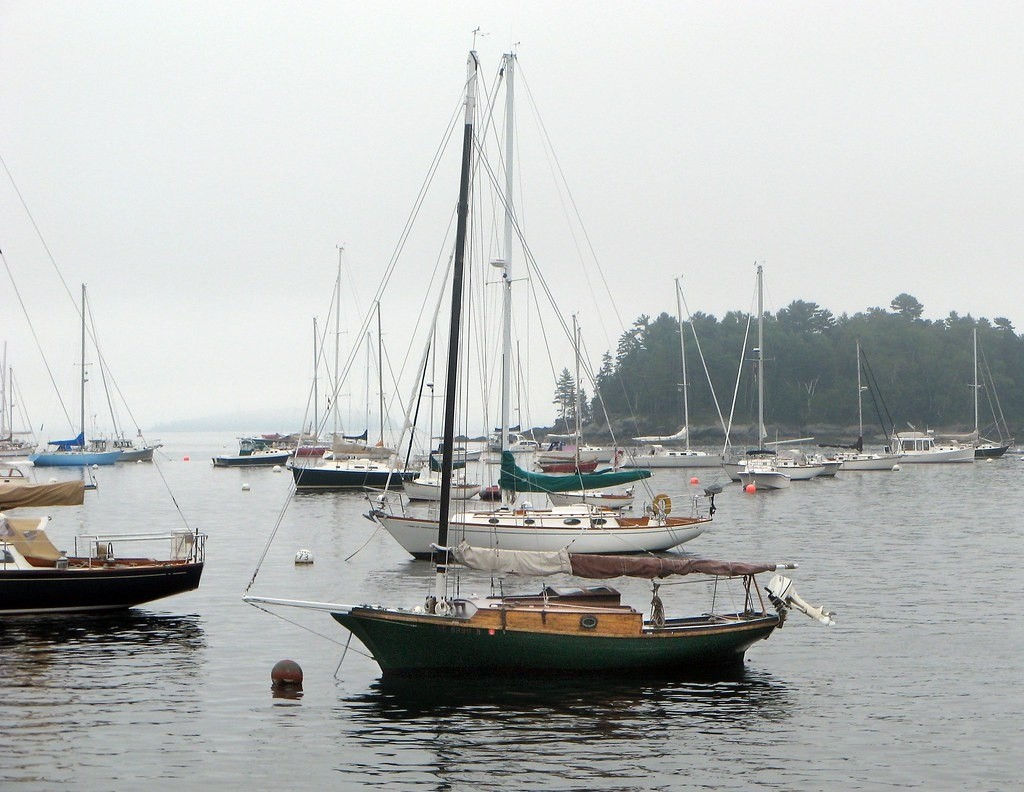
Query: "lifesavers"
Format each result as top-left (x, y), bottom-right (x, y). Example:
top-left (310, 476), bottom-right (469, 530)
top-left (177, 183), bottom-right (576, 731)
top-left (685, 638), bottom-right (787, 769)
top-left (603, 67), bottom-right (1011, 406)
top-left (653, 494), bottom-right (672, 515)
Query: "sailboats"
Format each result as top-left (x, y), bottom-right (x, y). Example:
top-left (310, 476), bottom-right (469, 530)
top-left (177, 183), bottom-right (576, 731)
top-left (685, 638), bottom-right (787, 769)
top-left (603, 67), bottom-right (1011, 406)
top-left (209, 28), bottom-right (1008, 681)
top-left (0, 279), bottom-right (168, 508)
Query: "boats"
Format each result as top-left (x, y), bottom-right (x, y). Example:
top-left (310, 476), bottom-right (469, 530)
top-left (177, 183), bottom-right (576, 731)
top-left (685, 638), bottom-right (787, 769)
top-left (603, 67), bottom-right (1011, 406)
top-left (0, 512), bottom-right (209, 624)
